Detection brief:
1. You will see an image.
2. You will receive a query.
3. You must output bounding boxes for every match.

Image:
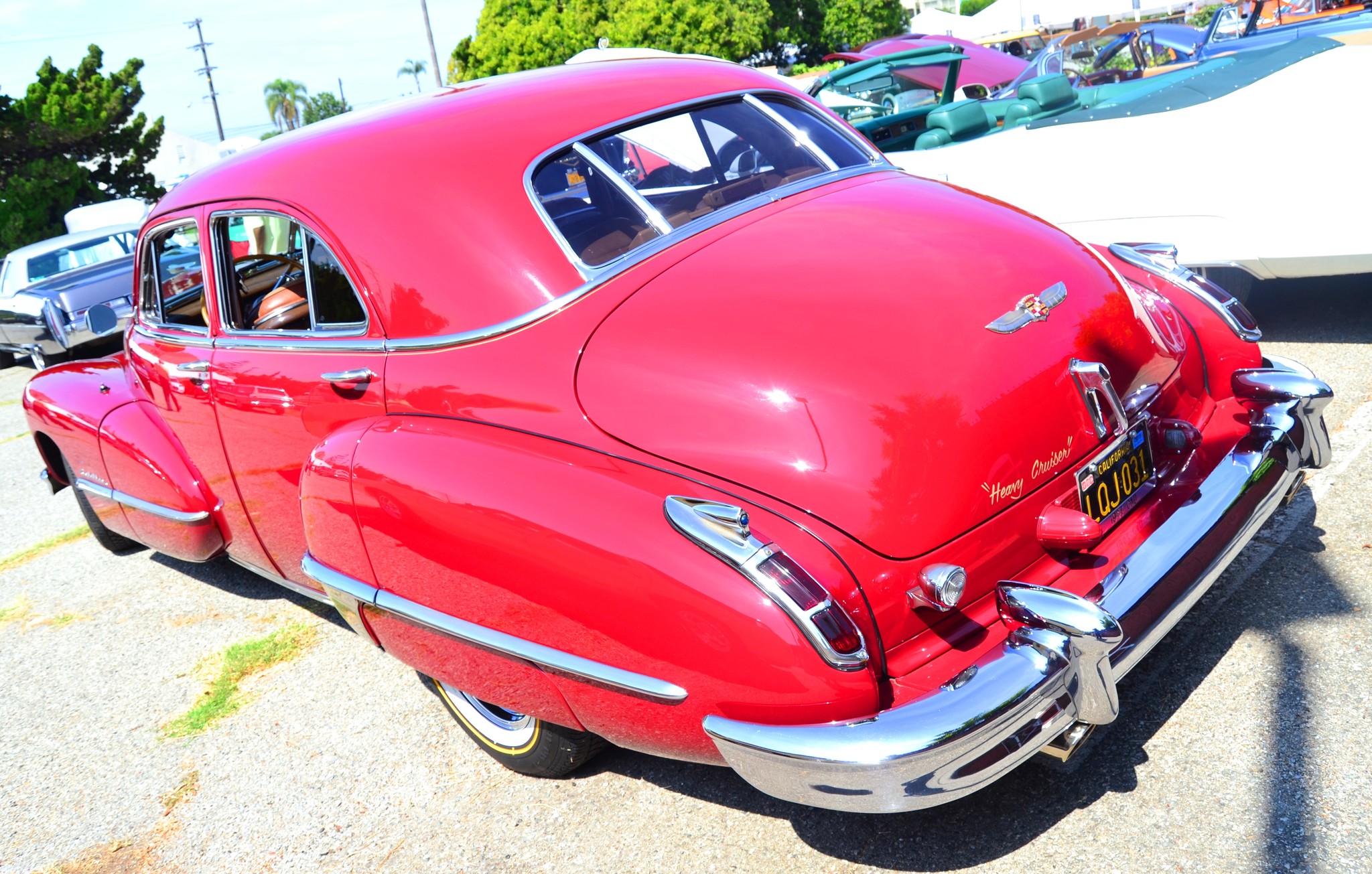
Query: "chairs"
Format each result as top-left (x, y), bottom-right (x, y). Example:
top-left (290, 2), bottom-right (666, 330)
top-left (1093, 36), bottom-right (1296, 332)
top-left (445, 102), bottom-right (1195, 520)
top-left (1003, 72), bottom-right (1081, 130)
top-left (914, 99), bottom-right (1003, 150)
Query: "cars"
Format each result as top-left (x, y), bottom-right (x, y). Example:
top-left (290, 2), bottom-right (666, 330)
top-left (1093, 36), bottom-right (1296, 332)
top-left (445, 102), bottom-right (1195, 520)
top-left (26, 51), bottom-right (1334, 821)
top-left (614, 1), bottom-right (1372, 301)
top-left (0, 222), bottom-right (206, 373)
